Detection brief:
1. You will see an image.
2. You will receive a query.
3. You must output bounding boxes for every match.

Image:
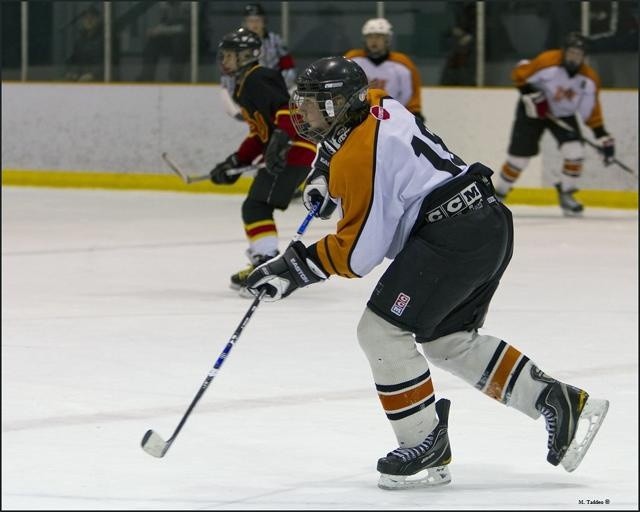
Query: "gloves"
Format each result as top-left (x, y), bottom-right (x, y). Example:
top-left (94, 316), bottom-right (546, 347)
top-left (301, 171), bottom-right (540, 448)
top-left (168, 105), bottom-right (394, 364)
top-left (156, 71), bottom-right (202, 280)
top-left (598, 133), bottom-right (614, 157)
top-left (532, 92), bottom-right (549, 117)
top-left (266, 129), bottom-right (291, 174)
top-left (210, 153), bottom-right (244, 185)
top-left (303, 145), bottom-right (338, 220)
top-left (248, 242), bottom-right (329, 300)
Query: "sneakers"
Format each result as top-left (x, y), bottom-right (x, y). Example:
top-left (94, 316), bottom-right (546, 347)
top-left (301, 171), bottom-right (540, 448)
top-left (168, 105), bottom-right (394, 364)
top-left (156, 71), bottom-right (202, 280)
top-left (231, 251), bottom-right (280, 283)
top-left (556, 182), bottom-right (581, 211)
top-left (530, 365), bottom-right (588, 465)
top-left (378, 399), bottom-right (451, 474)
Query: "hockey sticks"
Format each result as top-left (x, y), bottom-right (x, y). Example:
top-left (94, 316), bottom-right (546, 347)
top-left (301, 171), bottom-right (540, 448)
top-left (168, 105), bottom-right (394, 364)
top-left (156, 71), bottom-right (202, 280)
top-left (162, 152), bottom-right (267, 184)
top-left (141, 200), bottom-right (320, 458)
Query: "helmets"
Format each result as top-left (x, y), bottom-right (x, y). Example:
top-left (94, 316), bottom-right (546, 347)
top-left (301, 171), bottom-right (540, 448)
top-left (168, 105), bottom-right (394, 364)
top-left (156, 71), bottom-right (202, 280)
top-left (362, 18), bottom-right (392, 36)
top-left (217, 26), bottom-right (262, 75)
top-left (566, 35), bottom-right (585, 51)
top-left (288, 55), bottom-right (370, 144)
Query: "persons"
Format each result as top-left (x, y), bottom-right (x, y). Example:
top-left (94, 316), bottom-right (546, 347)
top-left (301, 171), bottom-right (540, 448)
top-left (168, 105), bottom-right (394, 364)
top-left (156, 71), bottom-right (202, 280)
top-left (343, 18), bottom-right (427, 128)
top-left (438, 4), bottom-right (481, 87)
top-left (221, 5), bottom-right (299, 119)
top-left (494, 32), bottom-right (616, 213)
top-left (209, 28), bottom-right (333, 297)
top-left (245, 57), bottom-right (609, 488)
top-left (60, 5), bottom-right (105, 83)
